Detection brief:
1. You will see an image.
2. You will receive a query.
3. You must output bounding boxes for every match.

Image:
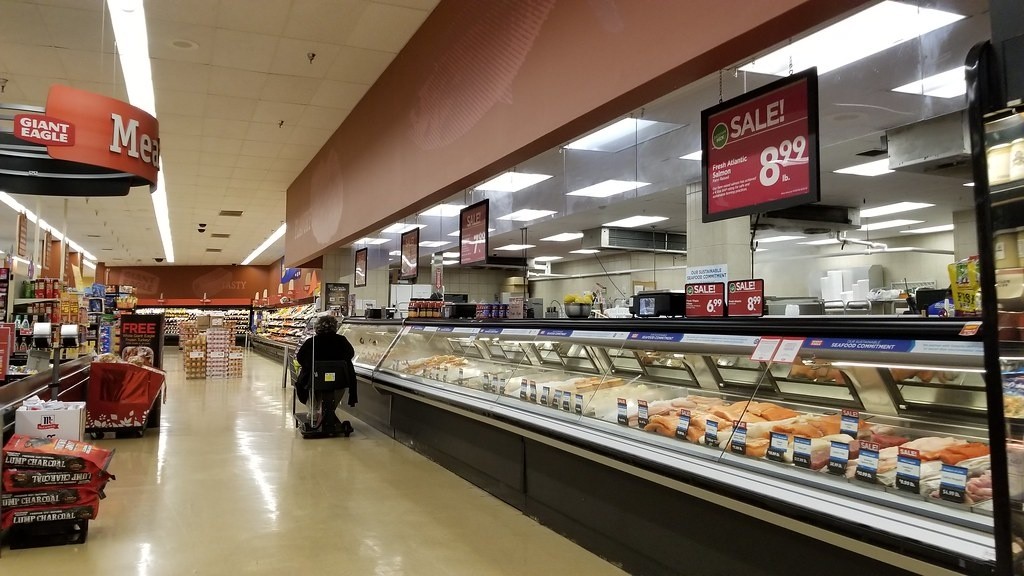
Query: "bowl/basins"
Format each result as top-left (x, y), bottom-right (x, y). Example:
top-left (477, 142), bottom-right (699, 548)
top-left (564, 302), bottom-right (591, 319)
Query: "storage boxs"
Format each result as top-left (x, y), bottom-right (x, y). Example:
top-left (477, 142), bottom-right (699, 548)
top-left (9, 518), bottom-right (89, 550)
top-left (22, 277), bottom-right (133, 354)
top-left (503, 276), bottom-right (528, 286)
top-left (178, 315), bottom-right (243, 380)
top-left (500, 284), bottom-right (528, 294)
top-left (14, 401), bottom-right (87, 443)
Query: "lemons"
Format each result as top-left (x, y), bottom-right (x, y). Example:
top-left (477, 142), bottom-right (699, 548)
top-left (563, 294), bottom-right (594, 303)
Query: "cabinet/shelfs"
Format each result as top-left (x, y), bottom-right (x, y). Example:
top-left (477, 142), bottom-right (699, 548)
top-left (262, 316), bottom-right (308, 337)
top-left (86, 296), bottom-right (107, 341)
top-left (105, 292), bottom-right (119, 312)
top-left (117, 291), bottom-right (135, 313)
top-left (164, 312), bottom-right (248, 335)
top-left (14, 297), bottom-right (60, 359)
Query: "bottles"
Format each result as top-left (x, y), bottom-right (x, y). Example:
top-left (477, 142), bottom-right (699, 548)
top-left (14, 314), bottom-right (60, 351)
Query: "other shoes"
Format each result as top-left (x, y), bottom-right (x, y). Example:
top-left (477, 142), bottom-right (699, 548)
top-left (307, 412), bottom-right (318, 421)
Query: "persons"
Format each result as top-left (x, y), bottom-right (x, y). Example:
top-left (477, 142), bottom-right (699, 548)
top-left (295, 314), bottom-right (358, 420)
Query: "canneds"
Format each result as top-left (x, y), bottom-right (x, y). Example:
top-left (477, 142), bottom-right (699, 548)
top-left (474, 302), bottom-right (509, 319)
top-left (986, 136), bottom-right (1024, 270)
